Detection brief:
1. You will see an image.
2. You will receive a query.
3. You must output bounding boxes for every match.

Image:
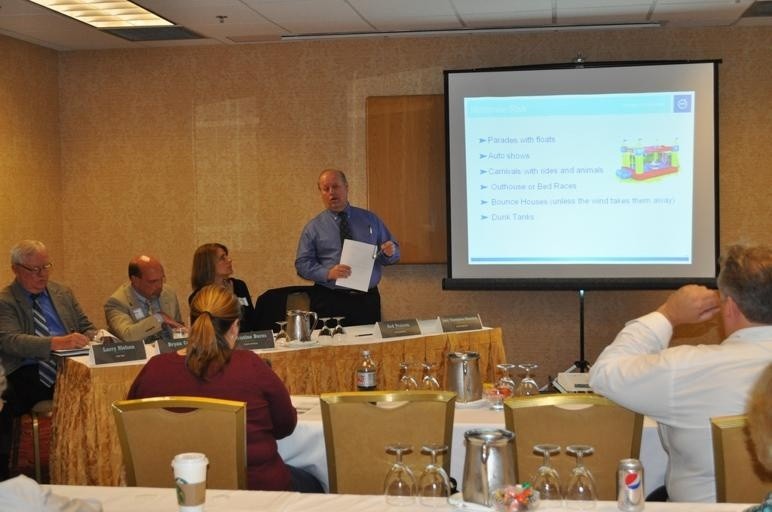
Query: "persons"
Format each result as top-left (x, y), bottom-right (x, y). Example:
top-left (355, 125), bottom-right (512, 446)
top-left (589, 248), bottom-right (772, 501)
top-left (0, 240), bottom-right (99, 478)
top-left (127, 284), bottom-right (323, 493)
top-left (295, 169), bottom-right (400, 332)
top-left (104, 253), bottom-right (189, 346)
top-left (187, 243), bottom-right (254, 333)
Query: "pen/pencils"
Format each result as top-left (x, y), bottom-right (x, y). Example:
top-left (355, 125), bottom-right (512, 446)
top-left (80, 327), bottom-right (90, 333)
top-left (355, 333), bottom-right (373, 337)
top-left (372, 249), bottom-right (382, 259)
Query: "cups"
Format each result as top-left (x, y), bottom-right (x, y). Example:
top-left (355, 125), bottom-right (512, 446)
top-left (489, 391), bottom-right (504, 409)
top-left (168, 454), bottom-right (211, 511)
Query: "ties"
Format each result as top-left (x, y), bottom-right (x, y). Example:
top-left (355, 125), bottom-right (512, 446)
top-left (337, 212), bottom-right (352, 247)
top-left (28, 294), bottom-right (57, 388)
top-left (145, 298), bottom-right (163, 341)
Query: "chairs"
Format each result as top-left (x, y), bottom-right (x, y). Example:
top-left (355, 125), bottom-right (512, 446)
top-left (707, 411), bottom-right (772, 503)
top-left (109, 395), bottom-right (250, 492)
top-left (501, 391), bottom-right (649, 500)
top-left (317, 387), bottom-right (463, 495)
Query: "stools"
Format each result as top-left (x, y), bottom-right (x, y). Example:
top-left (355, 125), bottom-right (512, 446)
top-left (0, 387), bottom-right (54, 484)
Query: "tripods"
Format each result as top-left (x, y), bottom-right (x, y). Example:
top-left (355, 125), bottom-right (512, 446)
top-left (537, 291), bottom-right (594, 393)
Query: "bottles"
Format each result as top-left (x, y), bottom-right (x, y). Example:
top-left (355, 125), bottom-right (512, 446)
top-left (357, 351), bottom-right (376, 391)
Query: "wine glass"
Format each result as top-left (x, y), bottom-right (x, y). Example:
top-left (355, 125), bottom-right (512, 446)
top-left (85, 329), bottom-right (105, 366)
top-left (172, 326), bottom-right (189, 338)
top-left (399, 359), bottom-right (540, 398)
top-left (275, 316), bottom-right (344, 343)
top-left (383, 444), bottom-right (603, 512)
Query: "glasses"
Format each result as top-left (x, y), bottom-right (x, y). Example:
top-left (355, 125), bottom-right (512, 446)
top-left (18, 263), bottom-right (54, 273)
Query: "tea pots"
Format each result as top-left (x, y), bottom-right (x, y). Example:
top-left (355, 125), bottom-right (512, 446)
top-left (288, 309), bottom-right (317, 344)
top-left (460, 428), bottom-right (516, 504)
top-left (443, 348), bottom-right (482, 402)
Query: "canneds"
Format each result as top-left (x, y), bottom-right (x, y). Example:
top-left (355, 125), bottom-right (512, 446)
top-left (617, 458), bottom-right (646, 512)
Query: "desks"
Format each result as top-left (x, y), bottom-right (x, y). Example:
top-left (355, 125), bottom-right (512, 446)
top-left (50, 312), bottom-right (507, 486)
top-left (273, 392), bottom-right (668, 503)
top-left (0, 483), bottom-right (772, 512)
